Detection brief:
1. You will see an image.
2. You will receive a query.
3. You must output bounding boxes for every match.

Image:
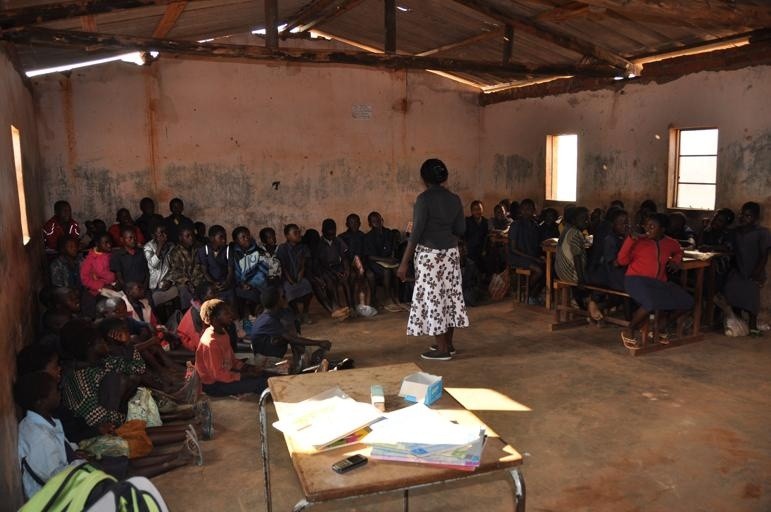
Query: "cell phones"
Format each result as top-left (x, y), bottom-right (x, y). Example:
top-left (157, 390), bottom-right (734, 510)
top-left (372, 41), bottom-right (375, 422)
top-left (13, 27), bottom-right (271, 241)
top-left (332, 454), bottom-right (368, 474)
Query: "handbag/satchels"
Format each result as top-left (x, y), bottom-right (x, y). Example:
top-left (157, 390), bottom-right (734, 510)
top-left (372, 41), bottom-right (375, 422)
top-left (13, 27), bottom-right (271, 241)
top-left (488, 266), bottom-right (511, 301)
top-left (126, 387), bottom-right (164, 429)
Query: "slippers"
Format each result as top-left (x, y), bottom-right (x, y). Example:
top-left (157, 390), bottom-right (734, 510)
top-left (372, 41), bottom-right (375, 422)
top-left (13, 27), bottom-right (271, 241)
top-left (383, 303), bottom-right (401, 313)
top-left (647, 331), bottom-right (670, 345)
top-left (398, 301), bottom-right (411, 313)
top-left (182, 369), bottom-right (211, 467)
top-left (620, 331), bottom-right (641, 350)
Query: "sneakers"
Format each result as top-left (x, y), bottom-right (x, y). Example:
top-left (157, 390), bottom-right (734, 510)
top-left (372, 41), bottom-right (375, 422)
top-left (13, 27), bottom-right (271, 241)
top-left (421, 349), bottom-right (453, 361)
top-left (429, 345), bottom-right (456, 355)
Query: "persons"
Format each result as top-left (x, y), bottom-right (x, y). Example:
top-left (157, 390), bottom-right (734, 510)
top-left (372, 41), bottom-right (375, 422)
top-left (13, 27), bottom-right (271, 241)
top-left (618, 213), bottom-right (694, 349)
top-left (398, 158), bottom-right (469, 359)
top-left (15, 321), bottom-right (214, 499)
top-left (41, 197), bottom-right (354, 396)
top-left (700, 201), bottom-right (769, 334)
top-left (462, 198), bottom-right (657, 321)
top-left (664, 212), bottom-right (695, 250)
top-left (276, 212), bottom-right (413, 323)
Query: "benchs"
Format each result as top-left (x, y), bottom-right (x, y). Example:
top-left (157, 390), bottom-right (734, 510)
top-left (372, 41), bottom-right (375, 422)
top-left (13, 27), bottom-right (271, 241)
top-left (552, 278), bottom-right (657, 347)
top-left (516, 266), bottom-right (535, 304)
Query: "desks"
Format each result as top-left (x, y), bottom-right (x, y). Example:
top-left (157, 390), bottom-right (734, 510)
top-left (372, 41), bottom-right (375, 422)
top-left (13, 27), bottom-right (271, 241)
top-left (546, 235), bottom-right (713, 340)
top-left (258, 361), bottom-right (530, 512)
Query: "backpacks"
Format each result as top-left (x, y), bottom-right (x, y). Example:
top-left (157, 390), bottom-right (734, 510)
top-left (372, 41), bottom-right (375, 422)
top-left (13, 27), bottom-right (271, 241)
top-left (16, 459), bottom-right (170, 512)
